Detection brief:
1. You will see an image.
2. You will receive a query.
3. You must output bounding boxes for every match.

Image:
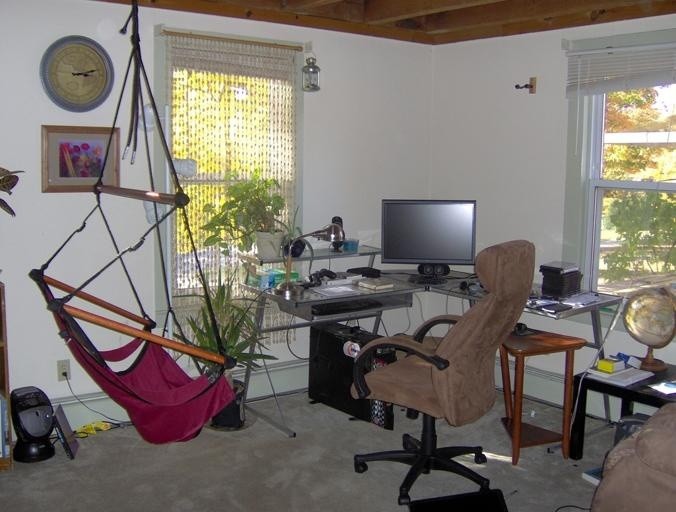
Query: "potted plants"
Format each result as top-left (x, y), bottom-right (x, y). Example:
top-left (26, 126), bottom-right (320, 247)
top-left (200, 168), bottom-right (284, 261)
top-left (173, 271), bottom-right (278, 428)
top-left (273, 206), bottom-right (315, 275)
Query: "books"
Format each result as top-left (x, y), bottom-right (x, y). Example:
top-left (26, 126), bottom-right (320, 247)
top-left (586, 367), bottom-right (654, 387)
top-left (358, 278), bottom-right (395, 291)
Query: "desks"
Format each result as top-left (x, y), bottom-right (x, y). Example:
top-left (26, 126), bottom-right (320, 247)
top-left (500, 329), bottom-right (587, 465)
top-left (570, 358), bottom-right (676, 459)
top-left (238, 269), bottom-right (623, 454)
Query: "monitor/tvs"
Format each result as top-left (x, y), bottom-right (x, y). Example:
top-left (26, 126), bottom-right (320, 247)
top-left (380, 199), bottom-right (476, 283)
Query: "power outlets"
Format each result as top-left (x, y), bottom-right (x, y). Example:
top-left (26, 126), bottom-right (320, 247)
top-left (58, 360), bottom-right (70, 381)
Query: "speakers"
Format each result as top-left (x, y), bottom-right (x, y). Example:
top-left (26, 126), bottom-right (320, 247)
top-left (10, 385), bottom-right (56, 463)
top-left (417, 265), bottom-right (451, 275)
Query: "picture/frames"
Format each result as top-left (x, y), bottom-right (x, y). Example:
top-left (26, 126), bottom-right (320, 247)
top-left (41, 125), bottom-right (120, 193)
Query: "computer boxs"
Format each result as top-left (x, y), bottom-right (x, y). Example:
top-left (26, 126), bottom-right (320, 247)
top-left (309, 322), bottom-right (394, 430)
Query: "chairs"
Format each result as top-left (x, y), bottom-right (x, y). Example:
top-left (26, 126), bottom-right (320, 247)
top-left (350, 240), bottom-right (535, 505)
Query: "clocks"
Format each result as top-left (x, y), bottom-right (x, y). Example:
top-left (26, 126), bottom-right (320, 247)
top-left (39, 35), bottom-right (114, 113)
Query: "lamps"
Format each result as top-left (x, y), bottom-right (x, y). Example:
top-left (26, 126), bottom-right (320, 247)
top-left (302, 58), bottom-right (320, 92)
top-left (272, 223), bottom-right (345, 296)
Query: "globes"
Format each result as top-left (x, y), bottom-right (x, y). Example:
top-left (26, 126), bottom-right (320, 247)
top-left (623, 287), bottom-right (676, 372)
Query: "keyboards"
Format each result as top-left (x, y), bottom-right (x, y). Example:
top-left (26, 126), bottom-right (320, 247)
top-left (312, 298), bottom-right (382, 314)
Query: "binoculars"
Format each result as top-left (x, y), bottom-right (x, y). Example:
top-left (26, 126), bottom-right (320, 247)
top-left (308, 268), bottom-right (337, 286)
